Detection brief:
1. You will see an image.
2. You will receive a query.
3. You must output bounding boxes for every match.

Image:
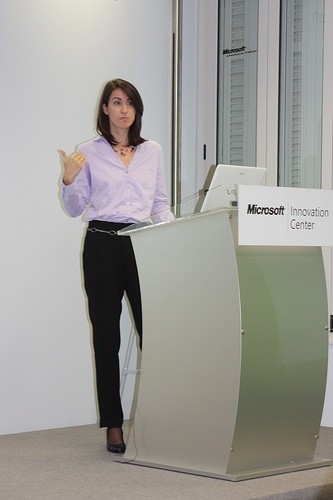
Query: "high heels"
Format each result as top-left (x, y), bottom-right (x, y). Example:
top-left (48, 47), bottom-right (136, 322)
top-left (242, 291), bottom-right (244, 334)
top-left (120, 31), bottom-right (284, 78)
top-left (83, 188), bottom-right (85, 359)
top-left (106, 427), bottom-right (126, 452)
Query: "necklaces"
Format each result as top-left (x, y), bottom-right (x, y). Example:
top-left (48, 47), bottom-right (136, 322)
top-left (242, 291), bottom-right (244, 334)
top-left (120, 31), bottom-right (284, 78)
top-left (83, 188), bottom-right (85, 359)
top-left (111, 145), bottom-right (135, 155)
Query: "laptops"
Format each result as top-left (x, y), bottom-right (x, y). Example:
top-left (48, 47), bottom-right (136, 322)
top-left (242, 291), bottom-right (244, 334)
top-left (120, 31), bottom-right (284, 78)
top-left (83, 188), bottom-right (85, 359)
top-left (200, 164), bottom-right (269, 213)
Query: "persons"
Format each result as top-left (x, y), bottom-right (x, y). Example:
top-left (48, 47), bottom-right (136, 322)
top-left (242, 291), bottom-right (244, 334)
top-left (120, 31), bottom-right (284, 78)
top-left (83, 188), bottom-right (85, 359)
top-left (58, 78), bottom-right (176, 454)
